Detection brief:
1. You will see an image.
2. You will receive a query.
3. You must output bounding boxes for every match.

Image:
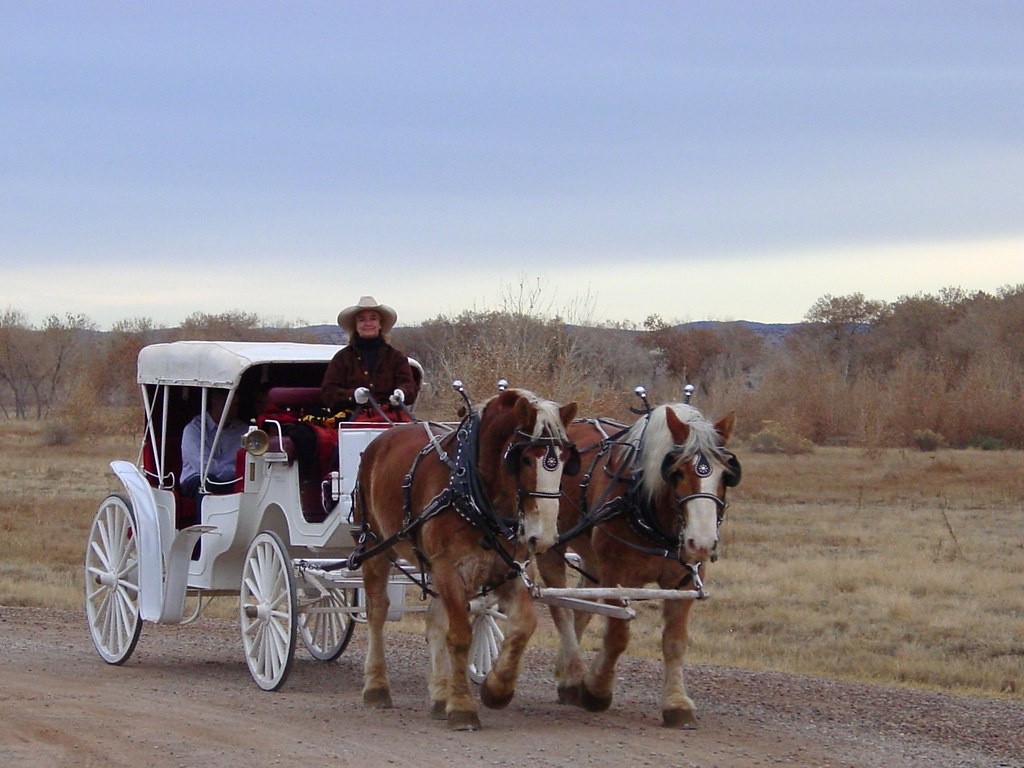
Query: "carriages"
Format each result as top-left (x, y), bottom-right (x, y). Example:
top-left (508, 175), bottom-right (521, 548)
top-left (81, 340), bottom-right (741, 733)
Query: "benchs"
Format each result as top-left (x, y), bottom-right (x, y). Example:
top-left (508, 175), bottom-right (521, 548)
top-left (143, 411), bottom-right (197, 517)
top-left (258, 387), bottom-right (328, 466)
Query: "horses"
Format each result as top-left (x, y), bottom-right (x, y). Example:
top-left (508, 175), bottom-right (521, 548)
top-left (354, 389), bottom-right (738, 731)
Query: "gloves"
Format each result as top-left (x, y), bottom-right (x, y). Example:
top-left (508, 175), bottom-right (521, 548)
top-left (354, 387), bottom-right (369, 404)
top-left (389, 389), bottom-right (405, 406)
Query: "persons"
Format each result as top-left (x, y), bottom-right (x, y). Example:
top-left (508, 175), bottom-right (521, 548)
top-left (178, 387), bottom-right (249, 560)
top-left (319, 295), bottom-right (418, 430)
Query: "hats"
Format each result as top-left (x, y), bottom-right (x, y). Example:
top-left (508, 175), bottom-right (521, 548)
top-left (337, 296), bottom-right (397, 336)
